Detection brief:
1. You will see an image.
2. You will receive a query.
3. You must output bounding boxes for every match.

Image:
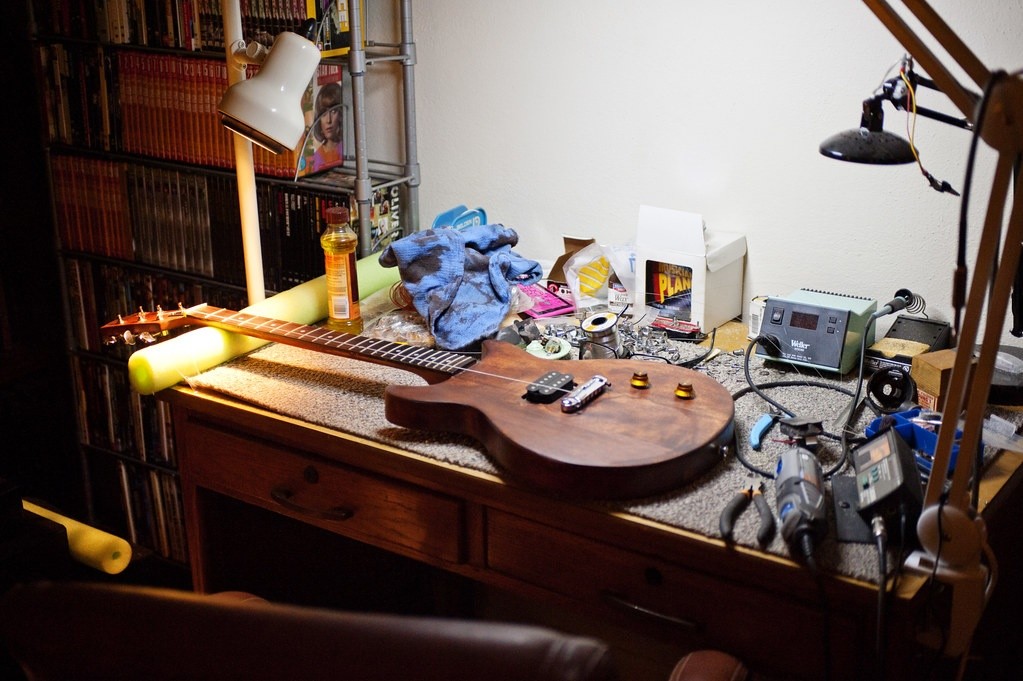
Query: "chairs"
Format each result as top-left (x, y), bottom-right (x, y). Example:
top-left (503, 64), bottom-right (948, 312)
top-left (0, 578), bottom-right (749, 681)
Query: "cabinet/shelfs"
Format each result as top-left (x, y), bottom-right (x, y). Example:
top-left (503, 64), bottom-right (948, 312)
top-left (24, 0), bottom-right (415, 594)
top-left (169, 401), bottom-right (877, 681)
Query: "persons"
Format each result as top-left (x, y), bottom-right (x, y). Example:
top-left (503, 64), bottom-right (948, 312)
top-left (315, 84), bottom-right (344, 172)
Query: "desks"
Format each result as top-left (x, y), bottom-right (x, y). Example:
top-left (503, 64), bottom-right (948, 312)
top-left (152, 238), bottom-right (1023, 681)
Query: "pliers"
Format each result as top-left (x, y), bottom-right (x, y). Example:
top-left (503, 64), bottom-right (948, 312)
top-left (749, 402), bottom-right (790, 450)
top-left (718, 471), bottom-right (775, 544)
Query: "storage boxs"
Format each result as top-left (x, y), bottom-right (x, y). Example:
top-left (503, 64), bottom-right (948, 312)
top-left (634, 204), bottom-right (746, 333)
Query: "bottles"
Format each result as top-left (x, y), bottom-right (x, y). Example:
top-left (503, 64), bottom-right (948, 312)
top-left (320, 207), bottom-right (364, 335)
top-left (608, 271), bottom-right (633, 318)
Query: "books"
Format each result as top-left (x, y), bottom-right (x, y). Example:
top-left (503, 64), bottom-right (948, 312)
top-left (23, 0), bottom-right (350, 180)
top-left (49, 151), bottom-right (401, 365)
top-left (73, 356), bottom-right (189, 565)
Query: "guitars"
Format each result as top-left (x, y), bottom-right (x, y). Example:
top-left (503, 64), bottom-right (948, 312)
top-left (100, 301), bottom-right (738, 500)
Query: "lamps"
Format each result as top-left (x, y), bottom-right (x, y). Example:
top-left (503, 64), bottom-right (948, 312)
top-left (818, 52), bottom-right (1022, 406)
top-left (216, 31), bottom-right (321, 155)
